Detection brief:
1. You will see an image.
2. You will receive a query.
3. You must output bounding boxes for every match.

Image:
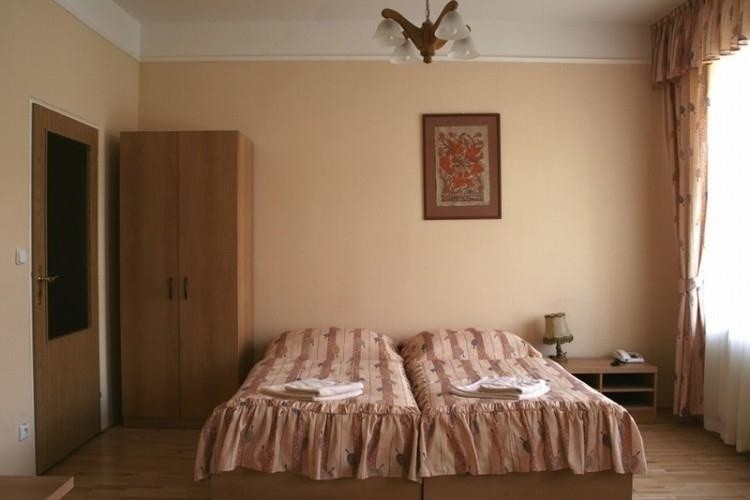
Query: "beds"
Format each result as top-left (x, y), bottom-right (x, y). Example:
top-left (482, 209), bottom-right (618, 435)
top-left (194, 327), bottom-right (423, 500)
top-left (399, 328), bottom-right (647, 500)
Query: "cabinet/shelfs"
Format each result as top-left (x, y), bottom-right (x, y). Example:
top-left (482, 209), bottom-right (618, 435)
top-left (119, 130), bottom-right (255, 428)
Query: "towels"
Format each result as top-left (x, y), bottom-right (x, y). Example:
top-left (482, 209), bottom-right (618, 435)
top-left (260, 378), bottom-right (364, 402)
top-left (447, 376), bottom-right (552, 401)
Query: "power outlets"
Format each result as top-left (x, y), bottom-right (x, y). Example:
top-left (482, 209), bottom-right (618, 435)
top-left (19, 423), bottom-right (29, 441)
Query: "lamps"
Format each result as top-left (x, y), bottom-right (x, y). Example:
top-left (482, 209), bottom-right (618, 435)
top-left (373, 0), bottom-right (479, 65)
top-left (543, 313), bottom-right (573, 363)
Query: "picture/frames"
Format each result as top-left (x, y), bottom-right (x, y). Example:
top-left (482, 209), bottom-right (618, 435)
top-left (423, 114), bottom-right (500, 220)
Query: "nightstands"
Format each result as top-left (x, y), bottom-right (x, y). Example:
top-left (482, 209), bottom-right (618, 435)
top-left (564, 357), bottom-right (658, 425)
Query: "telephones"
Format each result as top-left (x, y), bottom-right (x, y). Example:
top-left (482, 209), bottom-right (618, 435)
top-left (612, 348), bottom-right (644, 363)
